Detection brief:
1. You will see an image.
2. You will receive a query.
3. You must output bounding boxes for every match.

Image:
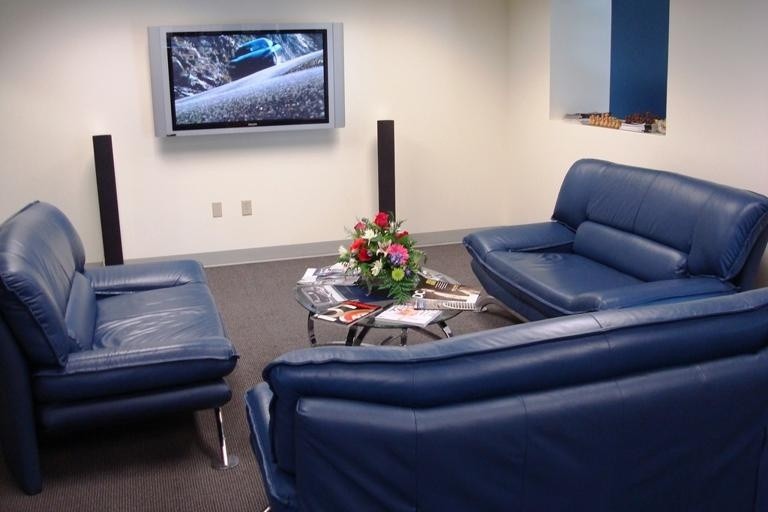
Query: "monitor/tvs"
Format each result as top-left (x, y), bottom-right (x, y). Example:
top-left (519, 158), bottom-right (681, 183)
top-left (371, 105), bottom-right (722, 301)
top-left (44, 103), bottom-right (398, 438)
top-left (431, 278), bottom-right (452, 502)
top-left (147, 22), bottom-right (345, 138)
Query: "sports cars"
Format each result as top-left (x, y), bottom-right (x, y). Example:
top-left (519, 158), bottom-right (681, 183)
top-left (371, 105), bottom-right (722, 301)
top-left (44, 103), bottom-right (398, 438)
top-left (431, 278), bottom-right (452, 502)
top-left (226, 36), bottom-right (283, 81)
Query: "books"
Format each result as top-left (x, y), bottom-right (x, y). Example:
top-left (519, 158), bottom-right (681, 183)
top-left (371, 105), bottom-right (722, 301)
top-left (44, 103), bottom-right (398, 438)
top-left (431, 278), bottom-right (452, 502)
top-left (617, 122), bottom-right (659, 132)
top-left (562, 112), bottom-right (610, 125)
top-left (297, 257), bottom-right (481, 329)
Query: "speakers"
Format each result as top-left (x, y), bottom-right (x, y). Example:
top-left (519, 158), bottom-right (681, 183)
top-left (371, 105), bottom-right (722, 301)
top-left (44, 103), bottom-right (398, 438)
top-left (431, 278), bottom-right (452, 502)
top-left (92, 134), bottom-right (124, 264)
top-left (377, 120), bottom-right (395, 218)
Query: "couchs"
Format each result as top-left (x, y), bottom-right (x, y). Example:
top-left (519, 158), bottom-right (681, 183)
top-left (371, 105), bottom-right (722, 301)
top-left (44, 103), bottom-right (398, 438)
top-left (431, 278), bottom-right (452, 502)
top-left (243, 287), bottom-right (766, 512)
top-left (0, 201), bottom-right (239, 495)
top-left (462, 159), bottom-right (767, 321)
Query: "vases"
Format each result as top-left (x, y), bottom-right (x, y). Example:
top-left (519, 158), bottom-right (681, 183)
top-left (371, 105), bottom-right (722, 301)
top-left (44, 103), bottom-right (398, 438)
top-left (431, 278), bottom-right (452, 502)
top-left (358, 276), bottom-right (390, 299)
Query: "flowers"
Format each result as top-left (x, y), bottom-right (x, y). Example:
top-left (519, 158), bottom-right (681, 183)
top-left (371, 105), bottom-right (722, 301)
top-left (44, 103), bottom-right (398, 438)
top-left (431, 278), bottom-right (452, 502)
top-left (337, 209), bottom-right (429, 306)
